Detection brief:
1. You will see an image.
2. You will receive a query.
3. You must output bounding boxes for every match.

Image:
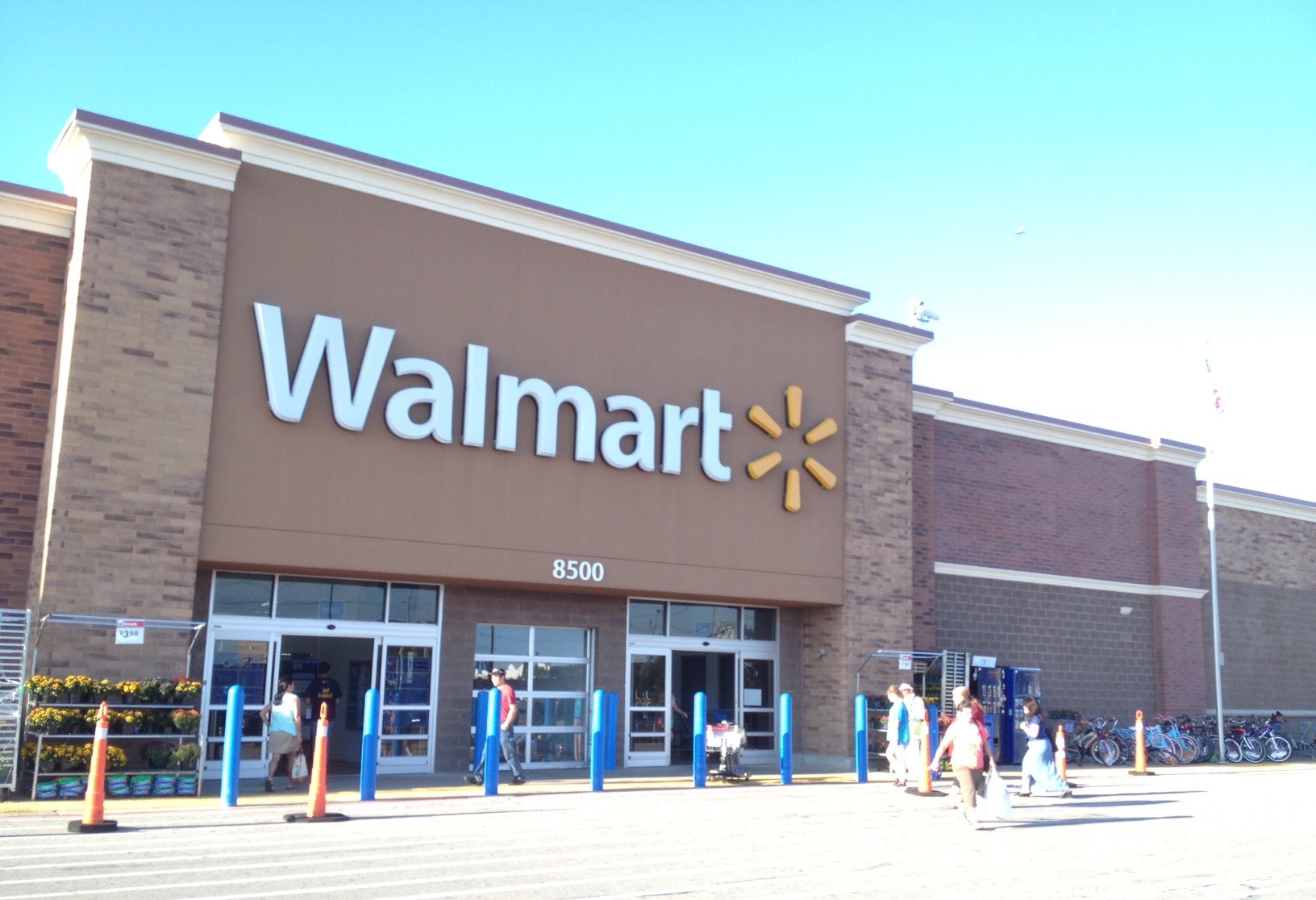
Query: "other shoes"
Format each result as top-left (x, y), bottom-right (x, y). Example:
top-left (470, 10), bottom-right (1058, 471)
top-left (264, 777), bottom-right (299, 792)
top-left (893, 777), bottom-right (1072, 832)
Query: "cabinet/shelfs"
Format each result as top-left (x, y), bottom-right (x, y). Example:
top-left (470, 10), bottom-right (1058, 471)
top-left (857, 649), bottom-right (947, 758)
top-left (14, 611), bottom-right (209, 798)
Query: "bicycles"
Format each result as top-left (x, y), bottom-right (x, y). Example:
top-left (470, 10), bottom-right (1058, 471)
top-left (1058, 714), bottom-right (1294, 767)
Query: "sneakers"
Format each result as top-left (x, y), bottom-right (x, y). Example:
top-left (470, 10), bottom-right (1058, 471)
top-left (464, 775), bottom-right (482, 787)
top-left (508, 776), bottom-right (525, 786)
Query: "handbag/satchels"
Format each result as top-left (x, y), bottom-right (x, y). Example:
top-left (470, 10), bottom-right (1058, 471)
top-left (982, 765), bottom-right (1013, 820)
top-left (291, 745), bottom-right (309, 783)
top-left (983, 754), bottom-right (990, 771)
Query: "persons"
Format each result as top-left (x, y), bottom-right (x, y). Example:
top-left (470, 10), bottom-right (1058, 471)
top-left (1269, 710), bottom-right (1301, 752)
top-left (462, 667), bottom-right (526, 787)
top-left (301, 662), bottom-right (342, 755)
top-left (385, 689), bottom-right (414, 760)
top-left (885, 685), bottom-right (912, 786)
top-left (950, 685), bottom-right (993, 807)
top-left (930, 699), bottom-right (997, 833)
top-left (655, 685), bottom-right (689, 741)
top-left (1014, 696), bottom-right (1074, 800)
top-left (896, 683), bottom-right (928, 782)
top-left (258, 676), bottom-right (305, 793)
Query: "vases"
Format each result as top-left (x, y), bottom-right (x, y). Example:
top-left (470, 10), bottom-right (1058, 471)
top-left (60, 759), bottom-right (77, 772)
top-left (105, 760), bottom-right (114, 772)
top-left (93, 692), bottom-right (107, 704)
top-left (176, 727), bottom-right (191, 735)
top-left (864, 689), bottom-right (955, 772)
top-left (40, 758), bottom-right (57, 773)
top-left (139, 724), bottom-right (150, 734)
top-left (25, 757), bottom-right (35, 769)
top-left (173, 696), bottom-right (184, 705)
top-left (120, 722), bottom-right (135, 735)
top-left (122, 694), bottom-right (136, 704)
top-left (69, 692), bottom-right (82, 704)
top-left (41, 688), bottom-right (58, 703)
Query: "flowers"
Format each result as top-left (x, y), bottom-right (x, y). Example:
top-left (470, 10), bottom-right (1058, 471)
top-left (170, 708), bottom-right (199, 729)
top-left (23, 674), bottom-right (65, 698)
top-left (89, 678), bottom-right (113, 694)
top-left (63, 674), bottom-right (93, 693)
top-left (105, 744), bottom-right (127, 767)
top-left (116, 708), bottom-right (145, 723)
top-left (57, 743), bottom-right (92, 763)
top-left (171, 676), bottom-right (202, 698)
top-left (35, 743), bottom-right (61, 762)
top-left (113, 679), bottom-right (139, 696)
top-left (20, 740), bottom-right (38, 759)
top-left (143, 710), bottom-right (155, 725)
top-left (25, 707), bottom-right (83, 733)
top-left (83, 707), bottom-right (119, 727)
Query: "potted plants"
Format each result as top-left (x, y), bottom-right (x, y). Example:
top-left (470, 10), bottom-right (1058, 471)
top-left (173, 741), bottom-right (203, 771)
top-left (141, 741), bottom-right (178, 769)
top-left (139, 676), bottom-right (158, 704)
top-left (154, 677), bottom-right (175, 704)
top-left (152, 708), bottom-right (172, 734)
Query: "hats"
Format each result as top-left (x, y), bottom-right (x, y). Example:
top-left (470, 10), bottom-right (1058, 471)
top-left (899, 683), bottom-right (913, 691)
top-left (488, 667), bottom-right (507, 675)
